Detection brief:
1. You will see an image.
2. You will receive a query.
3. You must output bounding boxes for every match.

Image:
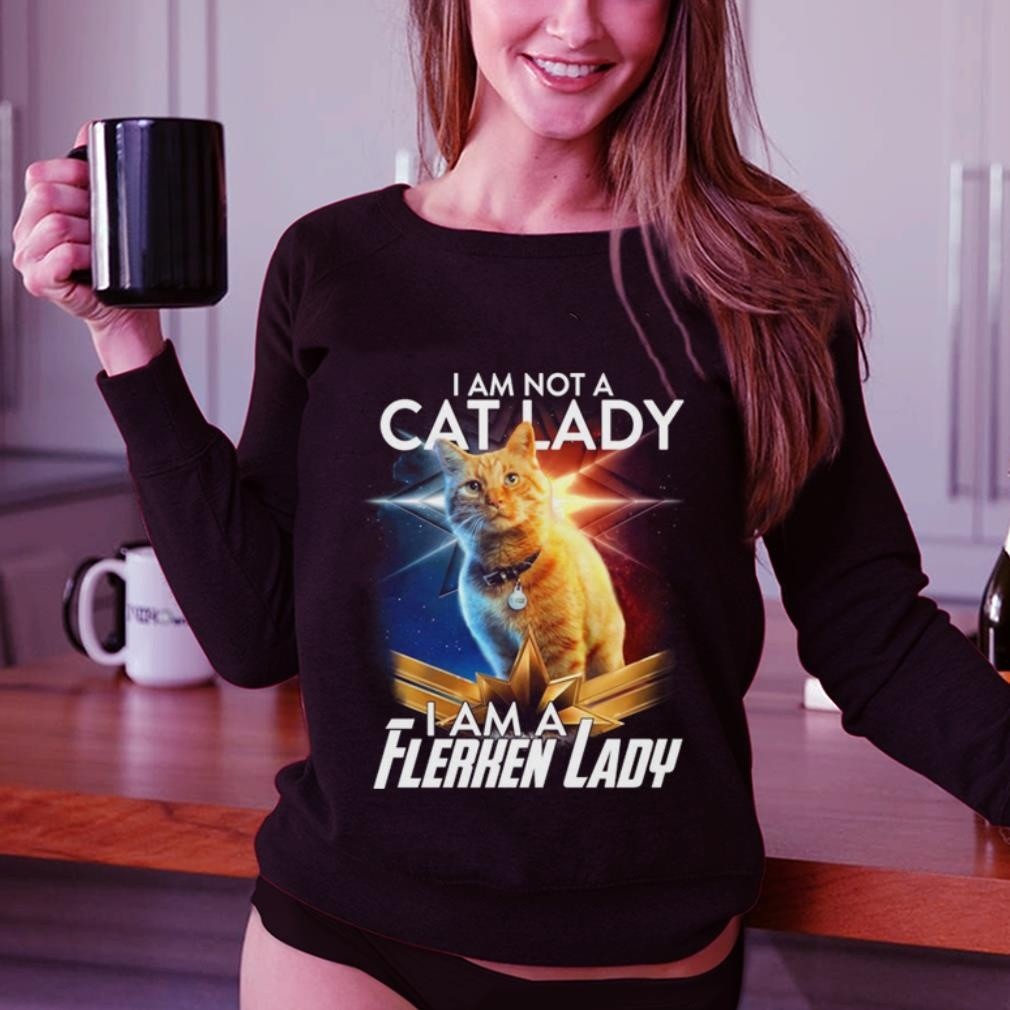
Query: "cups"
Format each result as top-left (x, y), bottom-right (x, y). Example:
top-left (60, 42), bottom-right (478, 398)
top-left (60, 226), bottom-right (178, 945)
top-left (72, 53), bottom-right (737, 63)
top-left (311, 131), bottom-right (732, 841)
top-left (62, 540), bottom-right (151, 681)
top-left (67, 117), bottom-right (229, 308)
top-left (78, 546), bottom-right (215, 687)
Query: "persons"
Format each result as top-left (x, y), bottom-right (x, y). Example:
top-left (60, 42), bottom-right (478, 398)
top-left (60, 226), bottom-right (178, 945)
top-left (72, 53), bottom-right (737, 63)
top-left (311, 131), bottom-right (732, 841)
top-left (12, 0), bottom-right (1010, 1010)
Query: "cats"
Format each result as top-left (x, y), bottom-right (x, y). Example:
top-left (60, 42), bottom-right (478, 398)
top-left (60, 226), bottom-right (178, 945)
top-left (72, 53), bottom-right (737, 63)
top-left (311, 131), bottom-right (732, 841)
top-left (433, 422), bottom-right (629, 685)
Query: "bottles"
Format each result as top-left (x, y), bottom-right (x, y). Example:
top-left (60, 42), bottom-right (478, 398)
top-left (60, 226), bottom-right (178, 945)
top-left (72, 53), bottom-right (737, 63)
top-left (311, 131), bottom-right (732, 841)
top-left (979, 527), bottom-right (1010, 671)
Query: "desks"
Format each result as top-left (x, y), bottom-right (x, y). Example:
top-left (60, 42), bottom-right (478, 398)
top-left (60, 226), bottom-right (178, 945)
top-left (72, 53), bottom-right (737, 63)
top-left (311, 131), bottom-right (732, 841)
top-left (1, 606), bottom-right (1010, 1010)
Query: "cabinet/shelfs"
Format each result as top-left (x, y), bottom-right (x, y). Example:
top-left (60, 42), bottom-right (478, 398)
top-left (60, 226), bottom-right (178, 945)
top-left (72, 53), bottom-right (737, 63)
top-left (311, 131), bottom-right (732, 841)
top-left (0, 0), bottom-right (1010, 593)
